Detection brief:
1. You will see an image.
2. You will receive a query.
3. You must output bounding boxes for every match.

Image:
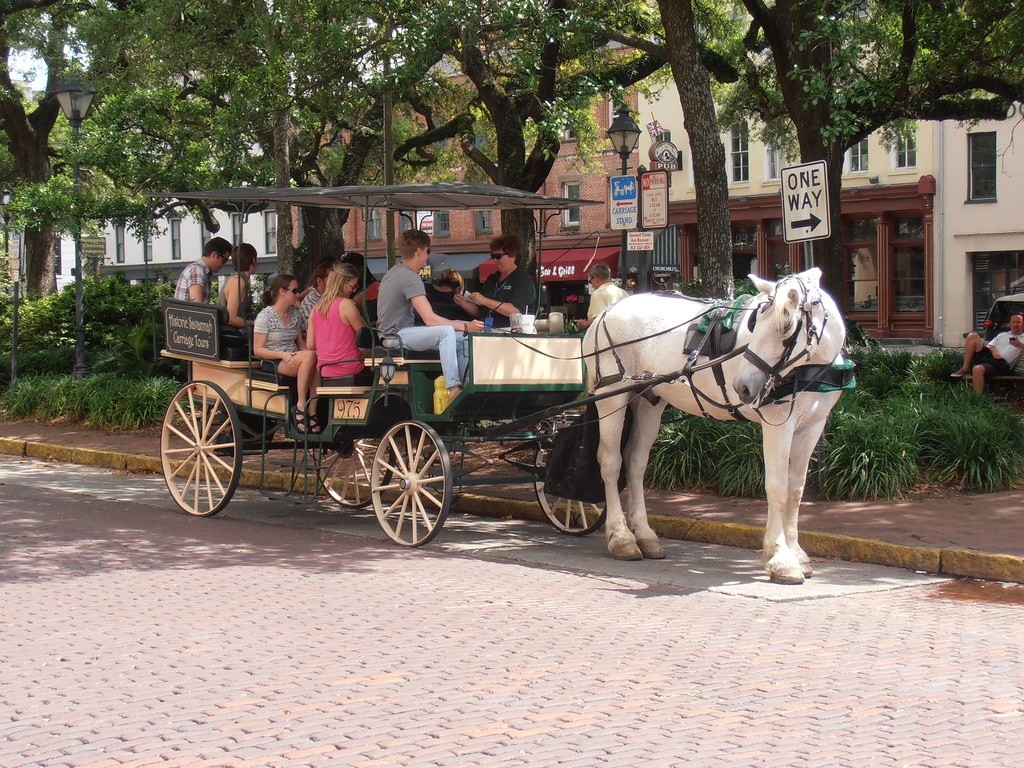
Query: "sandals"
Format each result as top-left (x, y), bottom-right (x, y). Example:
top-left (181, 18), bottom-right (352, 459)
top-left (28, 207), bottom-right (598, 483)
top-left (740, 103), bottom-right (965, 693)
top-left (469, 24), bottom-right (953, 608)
top-left (308, 410), bottom-right (321, 434)
top-left (292, 405), bottom-right (309, 434)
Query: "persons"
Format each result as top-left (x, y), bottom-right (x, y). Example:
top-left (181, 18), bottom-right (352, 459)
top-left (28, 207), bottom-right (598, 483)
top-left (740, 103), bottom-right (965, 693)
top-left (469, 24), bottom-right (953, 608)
top-left (305, 263), bottom-right (373, 386)
top-left (374, 230), bottom-right (472, 401)
top-left (173, 236), bottom-right (231, 304)
top-left (299, 261), bottom-right (333, 339)
top-left (251, 273), bottom-right (321, 433)
top-left (572, 262), bottom-right (630, 330)
top-left (452, 233), bottom-right (537, 334)
top-left (216, 241), bottom-right (258, 339)
top-left (342, 251), bottom-right (380, 348)
top-left (411, 266), bottom-right (469, 327)
top-left (949, 314), bottom-right (1024, 394)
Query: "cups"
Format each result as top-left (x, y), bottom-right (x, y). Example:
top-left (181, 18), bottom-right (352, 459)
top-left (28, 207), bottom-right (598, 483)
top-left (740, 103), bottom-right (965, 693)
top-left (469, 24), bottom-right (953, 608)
top-left (510, 314), bottom-right (522, 333)
top-left (520, 315), bottom-right (534, 333)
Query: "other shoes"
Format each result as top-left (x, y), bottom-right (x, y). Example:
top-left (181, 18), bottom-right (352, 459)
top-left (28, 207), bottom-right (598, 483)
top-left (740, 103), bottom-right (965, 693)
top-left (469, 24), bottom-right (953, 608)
top-left (950, 368), bottom-right (971, 377)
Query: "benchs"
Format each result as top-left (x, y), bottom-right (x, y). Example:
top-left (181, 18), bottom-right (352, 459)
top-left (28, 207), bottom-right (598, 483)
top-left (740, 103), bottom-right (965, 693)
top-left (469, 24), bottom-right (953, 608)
top-left (160, 298), bottom-right (254, 361)
top-left (961, 372), bottom-right (1024, 397)
top-left (245, 361), bottom-right (297, 391)
top-left (364, 346), bottom-right (442, 368)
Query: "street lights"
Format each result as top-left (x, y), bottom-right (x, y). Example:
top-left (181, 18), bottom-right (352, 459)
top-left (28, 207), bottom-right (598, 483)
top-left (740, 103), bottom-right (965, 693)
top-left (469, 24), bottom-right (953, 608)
top-left (51, 69), bottom-right (100, 379)
top-left (607, 100), bottom-right (644, 298)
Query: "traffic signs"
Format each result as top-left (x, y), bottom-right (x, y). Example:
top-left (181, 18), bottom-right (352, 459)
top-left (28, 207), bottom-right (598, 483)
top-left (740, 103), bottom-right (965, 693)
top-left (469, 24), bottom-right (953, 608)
top-left (781, 160), bottom-right (831, 243)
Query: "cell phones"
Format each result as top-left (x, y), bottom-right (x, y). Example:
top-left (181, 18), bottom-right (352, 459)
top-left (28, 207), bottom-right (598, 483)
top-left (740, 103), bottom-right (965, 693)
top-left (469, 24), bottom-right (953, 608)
top-left (1009, 337), bottom-right (1015, 342)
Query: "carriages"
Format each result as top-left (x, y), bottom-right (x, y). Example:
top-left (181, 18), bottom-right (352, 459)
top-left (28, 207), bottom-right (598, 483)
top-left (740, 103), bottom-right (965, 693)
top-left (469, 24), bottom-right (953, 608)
top-left (140, 177), bottom-right (859, 586)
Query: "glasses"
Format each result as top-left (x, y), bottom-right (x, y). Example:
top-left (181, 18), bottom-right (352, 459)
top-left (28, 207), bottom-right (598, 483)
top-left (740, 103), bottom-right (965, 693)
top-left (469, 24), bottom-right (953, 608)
top-left (588, 276), bottom-right (599, 284)
top-left (416, 248), bottom-right (431, 255)
top-left (214, 252), bottom-right (228, 263)
top-left (347, 282), bottom-right (356, 290)
top-left (490, 253), bottom-right (507, 259)
top-left (284, 288), bottom-right (300, 294)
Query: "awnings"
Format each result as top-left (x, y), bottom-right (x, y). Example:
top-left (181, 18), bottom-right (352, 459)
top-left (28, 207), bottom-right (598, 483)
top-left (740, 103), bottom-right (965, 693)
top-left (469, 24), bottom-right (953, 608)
top-left (430, 254), bottom-right (491, 278)
top-left (478, 247), bottom-right (621, 283)
top-left (366, 254), bottom-right (444, 282)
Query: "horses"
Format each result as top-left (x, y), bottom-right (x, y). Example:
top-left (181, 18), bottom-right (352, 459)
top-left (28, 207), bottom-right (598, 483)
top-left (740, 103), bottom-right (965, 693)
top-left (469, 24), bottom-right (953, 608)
top-left (582, 274), bottom-right (847, 585)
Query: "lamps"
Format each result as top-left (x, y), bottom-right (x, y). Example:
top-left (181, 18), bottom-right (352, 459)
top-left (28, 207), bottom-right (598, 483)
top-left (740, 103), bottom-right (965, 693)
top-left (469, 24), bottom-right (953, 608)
top-left (868, 176), bottom-right (879, 184)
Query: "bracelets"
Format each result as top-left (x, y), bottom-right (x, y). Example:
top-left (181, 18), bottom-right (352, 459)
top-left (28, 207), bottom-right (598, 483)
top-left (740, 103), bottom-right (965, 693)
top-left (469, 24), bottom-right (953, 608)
top-left (463, 323), bottom-right (468, 331)
